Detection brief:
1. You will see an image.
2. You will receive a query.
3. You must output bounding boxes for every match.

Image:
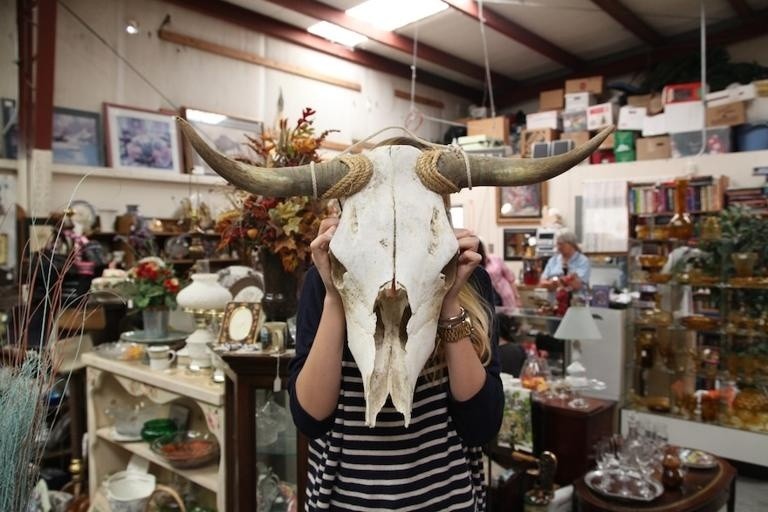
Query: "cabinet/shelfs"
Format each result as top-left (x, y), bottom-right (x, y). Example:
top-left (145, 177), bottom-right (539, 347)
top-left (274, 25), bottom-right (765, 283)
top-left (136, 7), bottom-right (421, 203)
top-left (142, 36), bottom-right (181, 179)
top-left (0, 208), bottom-right (310, 512)
top-left (530, 388), bottom-right (617, 479)
top-left (514, 251), bottom-right (627, 291)
top-left (623, 180), bottom-right (768, 467)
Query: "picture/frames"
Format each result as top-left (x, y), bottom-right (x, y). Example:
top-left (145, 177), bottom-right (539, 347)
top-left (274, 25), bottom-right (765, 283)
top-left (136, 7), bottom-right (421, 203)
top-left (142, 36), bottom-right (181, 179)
top-left (49, 100), bottom-right (266, 180)
top-left (495, 180), bottom-right (548, 226)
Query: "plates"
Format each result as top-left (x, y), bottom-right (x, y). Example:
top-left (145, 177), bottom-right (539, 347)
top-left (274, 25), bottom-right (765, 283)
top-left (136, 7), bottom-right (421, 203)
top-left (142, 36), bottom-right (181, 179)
top-left (91, 330), bottom-right (191, 361)
top-left (67, 200), bottom-right (97, 231)
top-left (678, 446), bottom-right (718, 469)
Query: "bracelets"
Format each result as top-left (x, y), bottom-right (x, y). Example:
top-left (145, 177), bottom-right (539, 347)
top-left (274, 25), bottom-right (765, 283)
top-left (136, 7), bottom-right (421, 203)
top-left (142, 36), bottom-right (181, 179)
top-left (439, 305), bottom-right (466, 323)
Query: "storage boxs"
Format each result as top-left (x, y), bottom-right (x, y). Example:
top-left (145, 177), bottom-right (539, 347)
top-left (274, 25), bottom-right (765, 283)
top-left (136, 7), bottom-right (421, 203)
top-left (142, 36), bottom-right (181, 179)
top-left (467, 74), bottom-right (768, 164)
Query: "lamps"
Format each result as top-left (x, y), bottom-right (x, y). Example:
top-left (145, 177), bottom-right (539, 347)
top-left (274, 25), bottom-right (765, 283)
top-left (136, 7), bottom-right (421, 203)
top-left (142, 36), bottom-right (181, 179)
top-left (554, 307), bottom-right (602, 372)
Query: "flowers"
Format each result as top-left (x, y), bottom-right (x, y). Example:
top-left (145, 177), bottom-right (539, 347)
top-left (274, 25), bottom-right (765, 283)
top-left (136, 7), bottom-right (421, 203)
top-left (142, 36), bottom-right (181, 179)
top-left (209, 107), bottom-right (341, 272)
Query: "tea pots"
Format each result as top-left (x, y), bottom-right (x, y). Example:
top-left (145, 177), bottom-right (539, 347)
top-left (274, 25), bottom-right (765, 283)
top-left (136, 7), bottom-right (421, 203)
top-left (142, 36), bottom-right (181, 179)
top-left (256, 462), bottom-right (280, 512)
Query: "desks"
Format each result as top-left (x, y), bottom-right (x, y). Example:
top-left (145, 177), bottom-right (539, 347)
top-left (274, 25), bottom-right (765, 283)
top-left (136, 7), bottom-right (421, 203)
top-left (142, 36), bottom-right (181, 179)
top-left (572, 445), bottom-right (737, 512)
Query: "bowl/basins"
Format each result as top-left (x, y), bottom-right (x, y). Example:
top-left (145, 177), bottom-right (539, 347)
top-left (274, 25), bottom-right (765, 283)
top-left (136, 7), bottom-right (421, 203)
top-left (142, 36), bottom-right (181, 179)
top-left (105, 400), bottom-right (220, 468)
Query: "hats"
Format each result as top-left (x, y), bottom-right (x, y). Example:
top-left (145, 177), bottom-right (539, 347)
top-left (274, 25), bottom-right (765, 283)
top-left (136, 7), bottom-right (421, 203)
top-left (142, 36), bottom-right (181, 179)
top-left (555, 229), bottom-right (578, 243)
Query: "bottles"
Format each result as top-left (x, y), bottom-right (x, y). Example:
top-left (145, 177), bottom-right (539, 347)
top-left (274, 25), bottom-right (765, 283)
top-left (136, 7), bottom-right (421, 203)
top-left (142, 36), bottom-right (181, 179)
top-left (176, 273), bottom-right (234, 312)
top-left (188, 238), bottom-right (206, 259)
top-left (118, 204), bottom-right (140, 268)
top-left (255, 400), bottom-right (290, 446)
top-left (519, 343), bottom-right (546, 389)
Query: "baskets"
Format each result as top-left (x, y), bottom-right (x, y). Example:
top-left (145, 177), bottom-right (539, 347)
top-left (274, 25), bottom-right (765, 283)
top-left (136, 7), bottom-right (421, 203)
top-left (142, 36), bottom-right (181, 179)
top-left (664, 215), bottom-right (693, 238)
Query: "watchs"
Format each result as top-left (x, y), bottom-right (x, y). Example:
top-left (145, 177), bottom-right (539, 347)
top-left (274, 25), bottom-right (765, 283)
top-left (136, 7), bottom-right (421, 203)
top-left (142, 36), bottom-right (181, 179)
top-left (436, 317), bottom-right (473, 341)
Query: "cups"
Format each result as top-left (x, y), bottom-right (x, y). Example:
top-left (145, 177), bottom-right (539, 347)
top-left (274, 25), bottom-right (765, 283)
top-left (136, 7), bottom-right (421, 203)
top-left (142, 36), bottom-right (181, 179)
top-left (143, 306), bottom-right (170, 338)
top-left (260, 322), bottom-right (288, 354)
top-left (146, 346), bottom-right (176, 371)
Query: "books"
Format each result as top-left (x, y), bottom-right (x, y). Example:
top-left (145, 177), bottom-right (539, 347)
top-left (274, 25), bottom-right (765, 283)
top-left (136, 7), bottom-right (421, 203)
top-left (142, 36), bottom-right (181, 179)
top-left (630, 176), bottom-right (720, 214)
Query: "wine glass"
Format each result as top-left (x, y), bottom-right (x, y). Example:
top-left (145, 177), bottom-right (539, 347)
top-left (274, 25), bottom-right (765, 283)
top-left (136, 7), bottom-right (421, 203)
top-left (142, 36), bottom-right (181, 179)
top-left (594, 420), bottom-right (668, 499)
top-left (544, 375), bottom-right (569, 399)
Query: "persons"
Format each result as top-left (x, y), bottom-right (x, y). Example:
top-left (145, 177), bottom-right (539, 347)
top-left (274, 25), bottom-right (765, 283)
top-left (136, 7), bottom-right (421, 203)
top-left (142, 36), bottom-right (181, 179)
top-left (497, 312), bottom-right (527, 377)
top-left (284, 134), bottom-right (504, 511)
top-left (461, 236), bottom-right (524, 308)
top-left (537, 227), bottom-right (591, 360)
